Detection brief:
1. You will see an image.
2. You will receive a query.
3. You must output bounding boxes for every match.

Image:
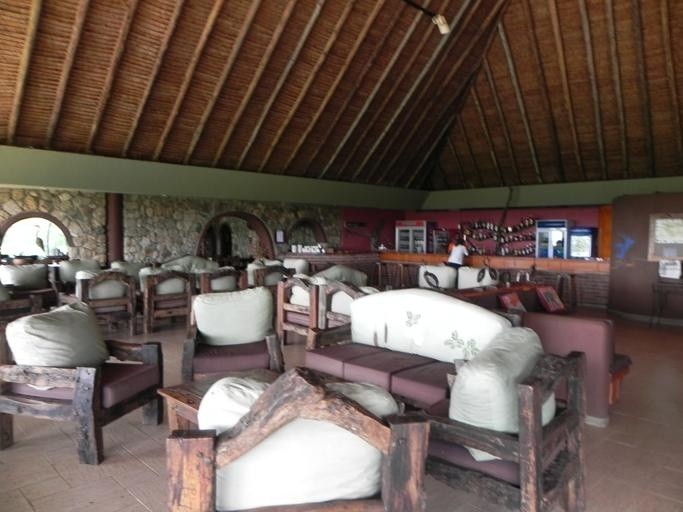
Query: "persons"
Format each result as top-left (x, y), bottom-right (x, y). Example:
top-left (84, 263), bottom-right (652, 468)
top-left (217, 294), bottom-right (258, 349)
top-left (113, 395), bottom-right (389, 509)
top-left (553, 240), bottom-right (563, 259)
top-left (446, 238), bottom-right (469, 289)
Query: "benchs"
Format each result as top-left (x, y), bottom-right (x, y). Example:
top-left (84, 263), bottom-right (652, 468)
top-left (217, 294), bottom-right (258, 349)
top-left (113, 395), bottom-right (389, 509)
top-left (454, 284), bottom-right (633, 427)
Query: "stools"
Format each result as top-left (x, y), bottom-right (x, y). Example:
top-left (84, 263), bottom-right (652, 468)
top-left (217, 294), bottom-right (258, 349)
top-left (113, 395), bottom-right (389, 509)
top-left (515, 271), bottom-right (531, 283)
top-left (500, 268), bottom-right (511, 282)
top-left (555, 274), bottom-right (573, 309)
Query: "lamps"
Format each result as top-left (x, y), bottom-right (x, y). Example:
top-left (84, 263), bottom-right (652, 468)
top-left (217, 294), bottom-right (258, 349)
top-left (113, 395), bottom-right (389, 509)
top-left (425, 10), bottom-right (450, 34)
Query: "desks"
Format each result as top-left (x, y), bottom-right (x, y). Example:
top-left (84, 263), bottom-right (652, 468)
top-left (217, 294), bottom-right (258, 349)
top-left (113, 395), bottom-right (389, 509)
top-left (649, 283), bottom-right (682, 328)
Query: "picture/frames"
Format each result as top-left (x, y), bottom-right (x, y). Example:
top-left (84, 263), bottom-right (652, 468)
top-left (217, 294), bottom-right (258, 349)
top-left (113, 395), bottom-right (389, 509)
top-left (275, 229), bottom-right (286, 244)
